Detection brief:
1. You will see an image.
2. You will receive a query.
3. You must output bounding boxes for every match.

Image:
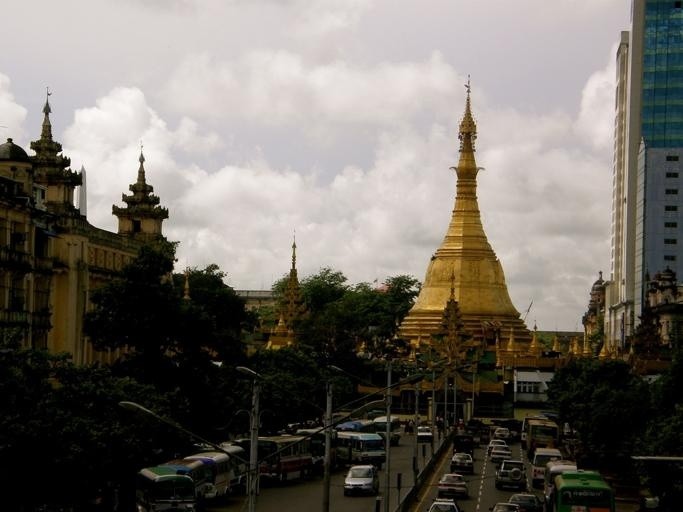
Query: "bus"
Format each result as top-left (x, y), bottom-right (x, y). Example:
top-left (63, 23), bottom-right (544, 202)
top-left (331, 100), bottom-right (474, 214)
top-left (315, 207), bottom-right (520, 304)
top-left (296, 427), bottom-right (386, 468)
top-left (521, 411), bottom-right (615, 512)
top-left (135, 436), bottom-right (312, 512)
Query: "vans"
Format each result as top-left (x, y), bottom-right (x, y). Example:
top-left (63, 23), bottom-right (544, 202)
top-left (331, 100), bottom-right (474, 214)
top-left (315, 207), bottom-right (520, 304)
top-left (374, 416), bottom-right (401, 444)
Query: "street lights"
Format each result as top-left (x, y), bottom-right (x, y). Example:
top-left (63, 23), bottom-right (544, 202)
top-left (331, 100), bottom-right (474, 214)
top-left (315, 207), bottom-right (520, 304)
top-left (630, 456), bottom-right (683, 460)
top-left (235, 363), bottom-right (426, 512)
top-left (118, 366), bottom-right (386, 512)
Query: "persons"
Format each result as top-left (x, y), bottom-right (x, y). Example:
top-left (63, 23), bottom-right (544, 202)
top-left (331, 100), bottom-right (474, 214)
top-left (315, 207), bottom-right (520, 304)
top-left (563, 422), bottom-right (578, 457)
top-left (458, 415), bottom-right (466, 431)
top-left (437, 418), bottom-right (443, 441)
top-left (405, 417), bottom-right (422, 434)
top-left (639, 488), bottom-right (661, 511)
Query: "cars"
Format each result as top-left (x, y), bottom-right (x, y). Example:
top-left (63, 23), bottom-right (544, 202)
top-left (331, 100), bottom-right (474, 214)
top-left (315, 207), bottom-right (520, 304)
top-left (344, 465), bottom-right (380, 498)
top-left (417, 426), bottom-right (434, 442)
top-left (508, 491), bottom-right (545, 512)
top-left (426, 427), bottom-right (526, 512)
